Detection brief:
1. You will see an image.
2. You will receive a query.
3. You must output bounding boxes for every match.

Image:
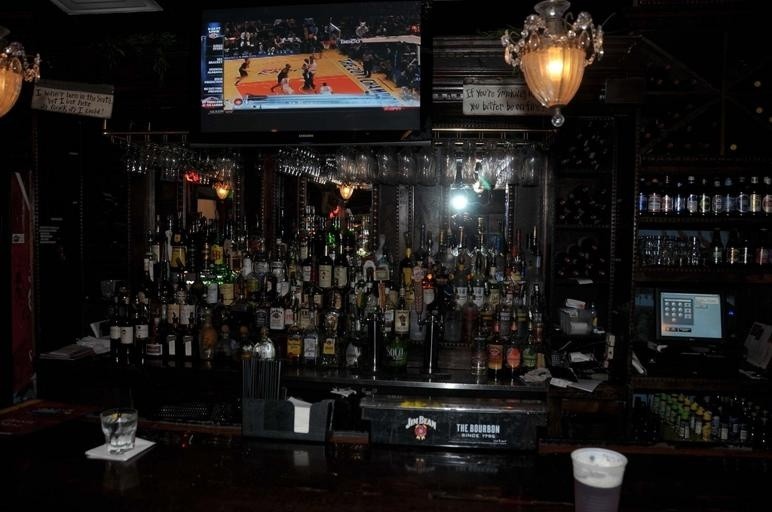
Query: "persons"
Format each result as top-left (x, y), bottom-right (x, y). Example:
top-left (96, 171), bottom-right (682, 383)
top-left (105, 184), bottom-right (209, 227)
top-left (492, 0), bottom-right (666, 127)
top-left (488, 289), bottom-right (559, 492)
top-left (319, 81), bottom-right (333, 94)
top-left (234, 56), bottom-right (250, 86)
top-left (350, 15), bottom-right (420, 96)
top-left (271, 63), bottom-right (297, 92)
top-left (225, 15), bottom-right (321, 58)
top-left (302, 55), bottom-right (315, 89)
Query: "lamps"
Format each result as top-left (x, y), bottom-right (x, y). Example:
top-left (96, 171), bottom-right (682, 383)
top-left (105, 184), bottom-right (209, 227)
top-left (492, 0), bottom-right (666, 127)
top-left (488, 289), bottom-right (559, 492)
top-left (0, 42), bottom-right (40, 120)
top-left (337, 184), bottom-right (355, 200)
top-left (501, 0), bottom-right (603, 127)
top-left (214, 181), bottom-right (230, 199)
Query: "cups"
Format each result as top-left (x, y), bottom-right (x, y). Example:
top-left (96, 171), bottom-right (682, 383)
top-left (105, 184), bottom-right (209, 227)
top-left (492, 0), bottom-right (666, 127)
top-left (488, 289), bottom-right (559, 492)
top-left (397, 147), bottom-right (415, 186)
top-left (572, 448), bottom-right (627, 512)
top-left (101, 409), bottom-right (137, 455)
top-left (435, 139), bottom-right (459, 187)
top-left (310, 153), bottom-right (336, 184)
top-left (459, 138), bottom-right (479, 187)
top-left (479, 137), bottom-right (500, 186)
top-left (375, 146), bottom-right (398, 185)
top-left (335, 146), bottom-right (355, 183)
top-left (355, 146), bottom-right (375, 182)
top-left (522, 138), bottom-right (543, 186)
top-left (415, 146), bottom-right (434, 185)
top-left (271, 145), bottom-right (321, 179)
top-left (500, 138), bottom-right (522, 184)
top-left (120, 144), bottom-right (240, 181)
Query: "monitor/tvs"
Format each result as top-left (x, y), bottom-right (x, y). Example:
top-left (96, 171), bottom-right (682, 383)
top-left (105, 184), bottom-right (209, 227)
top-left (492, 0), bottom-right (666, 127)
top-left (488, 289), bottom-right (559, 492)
top-left (653, 289), bottom-right (730, 357)
top-left (191, 0), bottom-right (434, 147)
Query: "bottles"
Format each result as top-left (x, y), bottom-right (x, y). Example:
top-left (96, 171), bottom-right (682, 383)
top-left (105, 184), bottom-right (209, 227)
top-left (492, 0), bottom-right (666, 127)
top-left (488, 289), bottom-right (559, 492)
top-left (636, 174), bottom-right (772, 266)
top-left (109, 211), bottom-right (543, 375)
top-left (647, 393), bottom-right (772, 473)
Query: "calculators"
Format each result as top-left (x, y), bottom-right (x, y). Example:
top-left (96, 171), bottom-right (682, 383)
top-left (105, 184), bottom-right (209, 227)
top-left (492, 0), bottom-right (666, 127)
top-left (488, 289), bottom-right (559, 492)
top-left (663, 296), bottom-right (694, 325)
